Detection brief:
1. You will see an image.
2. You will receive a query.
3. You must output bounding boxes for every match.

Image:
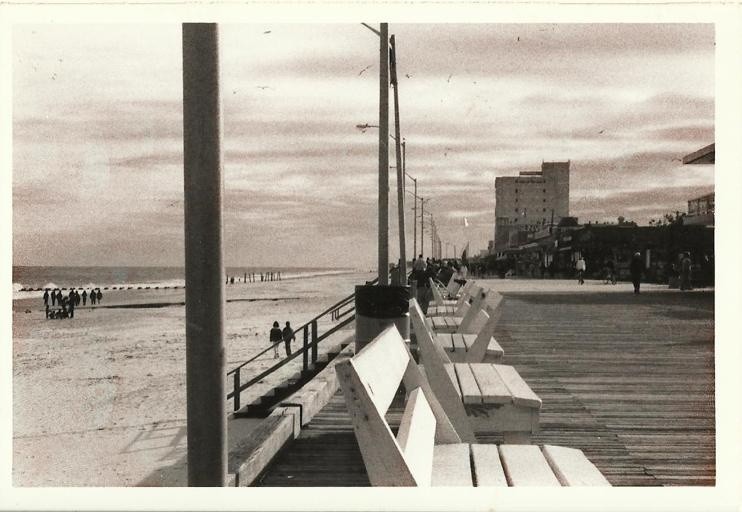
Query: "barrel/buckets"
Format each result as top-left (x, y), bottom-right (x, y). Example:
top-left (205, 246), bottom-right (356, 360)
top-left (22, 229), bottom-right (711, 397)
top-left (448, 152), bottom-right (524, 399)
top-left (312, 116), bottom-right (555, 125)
top-left (354, 285), bottom-right (411, 392)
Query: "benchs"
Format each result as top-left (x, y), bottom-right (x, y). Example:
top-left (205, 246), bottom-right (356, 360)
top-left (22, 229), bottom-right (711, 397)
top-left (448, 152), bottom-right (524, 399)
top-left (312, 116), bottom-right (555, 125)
top-left (344, 322), bottom-right (612, 486)
top-left (407, 264), bottom-right (543, 444)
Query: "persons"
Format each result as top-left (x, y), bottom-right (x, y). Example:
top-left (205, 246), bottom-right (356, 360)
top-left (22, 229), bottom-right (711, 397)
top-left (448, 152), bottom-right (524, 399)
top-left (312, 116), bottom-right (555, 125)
top-left (42, 286), bottom-right (103, 320)
top-left (407, 253), bottom-right (513, 301)
top-left (389, 263), bottom-right (400, 286)
top-left (630, 251), bottom-right (647, 295)
top-left (678, 250), bottom-right (693, 291)
top-left (575, 256), bottom-right (586, 285)
top-left (606, 257), bottom-right (618, 281)
top-left (282, 320), bottom-right (296, 357)
top-left (269, 321), bottom-right (282, 358)
